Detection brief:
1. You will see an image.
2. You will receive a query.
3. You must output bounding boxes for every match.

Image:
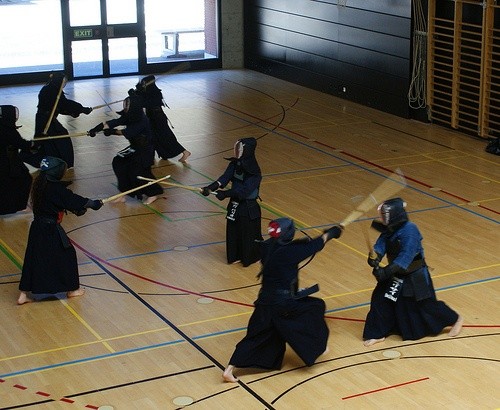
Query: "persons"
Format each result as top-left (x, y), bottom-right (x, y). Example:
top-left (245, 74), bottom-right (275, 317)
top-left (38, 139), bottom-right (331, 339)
top-left (1, 104), bottom-right (43, 218)
top-left (199, 137), bottom-right (266, 265)
top-left (88, 95), bottom-right (165, 206)
top-left (220, 216), bottom-right (329, 384)
top-left (361, 196), bottom-right (463, 348)
top-left (127, 76), bottom-right (192, 163)
top-left (31, 71), bottom-right (95, 169)
top-left (15, 157), bottom-right (104, 306)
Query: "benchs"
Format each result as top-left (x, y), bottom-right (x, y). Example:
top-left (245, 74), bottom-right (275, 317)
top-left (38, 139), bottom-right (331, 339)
top-left (160, 29), bottom-right (205, 58)
